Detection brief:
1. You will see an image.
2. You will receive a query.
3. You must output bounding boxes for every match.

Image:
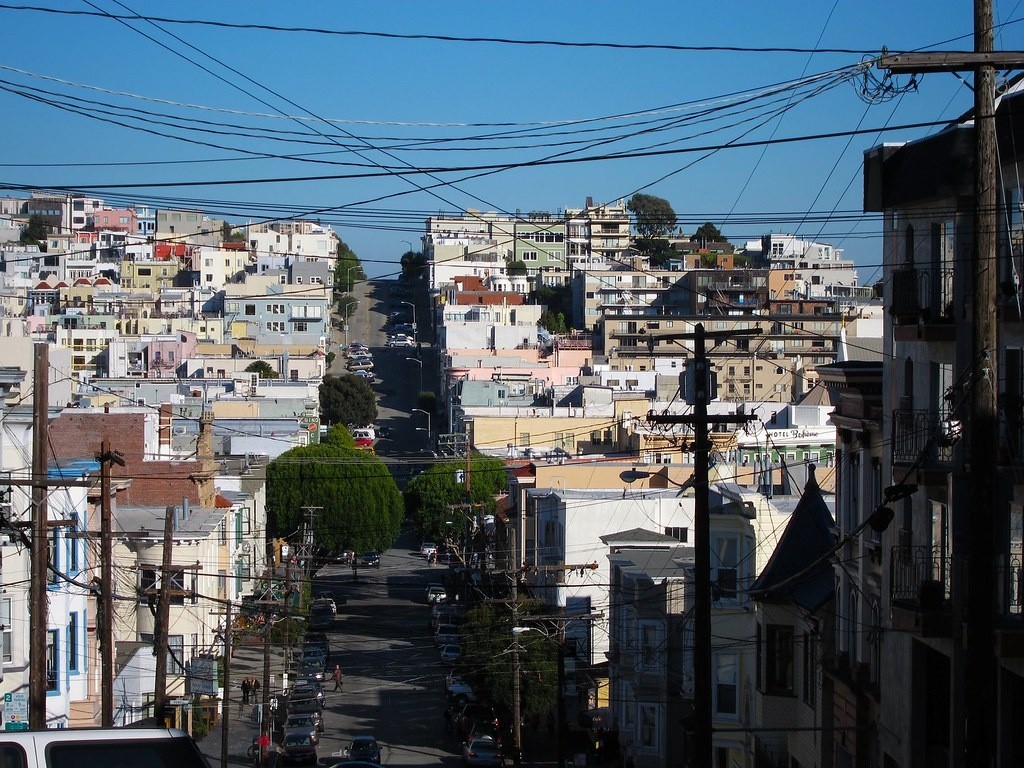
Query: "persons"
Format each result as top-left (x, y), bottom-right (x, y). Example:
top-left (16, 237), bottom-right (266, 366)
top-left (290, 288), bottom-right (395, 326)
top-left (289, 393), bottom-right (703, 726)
top-left (241, 675), bottom-right (260, 706)
top-left (428, 550), bottom-right (437, 567)
top-left (331, 665), bottom-right (344, 693)
top-left (290, 553), bottom-right (297, 565)
top-left (257, 612), bottom-right (266, 630)
top-left (346, 550), bottom-right (358, 576)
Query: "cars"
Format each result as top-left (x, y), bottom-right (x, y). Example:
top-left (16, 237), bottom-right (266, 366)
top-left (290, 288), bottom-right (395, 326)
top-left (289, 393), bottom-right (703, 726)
top-left (352, 550), bottom-right (380, 569)
top-left (344, 343), bottom-right (375, 385)
top-left (388, 298), bottom-right (414, 348)
top-left (280, 597), bottom-right (384, 768)
top-left (424, 582), bottom-right (505, 768)
top-left (350, 427), bottom-right (376, 456)
top-left (420, 543), bottom-right (438, 555)
top-left (378, 427), bottom-right (390, 438)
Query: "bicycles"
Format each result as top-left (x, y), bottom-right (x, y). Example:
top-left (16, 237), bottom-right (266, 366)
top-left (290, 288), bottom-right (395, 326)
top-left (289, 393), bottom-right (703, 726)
top-left (247, 736), bottom-right (259, 767)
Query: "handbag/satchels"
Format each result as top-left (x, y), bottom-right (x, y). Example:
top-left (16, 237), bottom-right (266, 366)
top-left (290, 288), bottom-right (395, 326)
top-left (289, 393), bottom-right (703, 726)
top-left (254, 680), bottom-right (260, 689)
top-left (340, 681), bottom-right (343, 685)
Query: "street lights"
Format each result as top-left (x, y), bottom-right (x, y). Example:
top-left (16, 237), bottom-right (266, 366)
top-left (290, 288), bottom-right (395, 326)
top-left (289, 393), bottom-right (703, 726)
top-left (406, 358), bottom-right (422, 393)
top-left (401, 301), bottom-right (415, 348)
top-left (512, 626), bottom-right (565, 768)
top-left (347, 265), bottom-right (360, 297)
top-left (345, 300), bottom-right (360, 350)
top-left (412, 409), bottom-right (430, 440)
top-left (620, 469), bottom-right (712, 768)
top-left (399, 240), bottom-right (412, 252)
